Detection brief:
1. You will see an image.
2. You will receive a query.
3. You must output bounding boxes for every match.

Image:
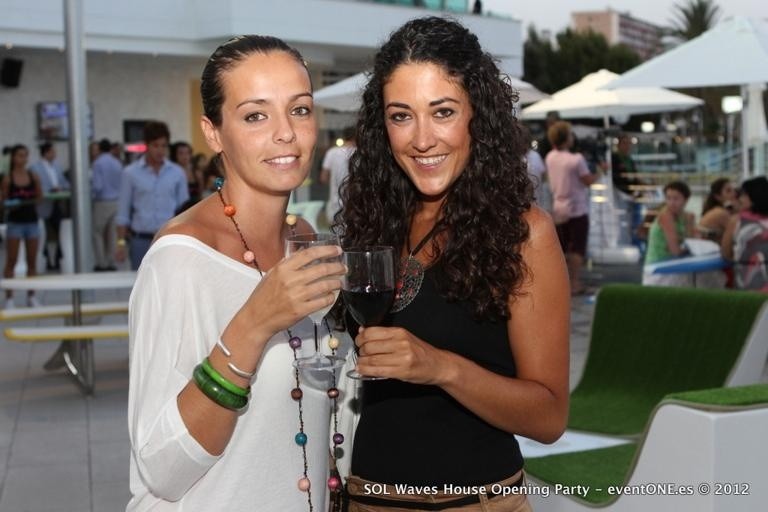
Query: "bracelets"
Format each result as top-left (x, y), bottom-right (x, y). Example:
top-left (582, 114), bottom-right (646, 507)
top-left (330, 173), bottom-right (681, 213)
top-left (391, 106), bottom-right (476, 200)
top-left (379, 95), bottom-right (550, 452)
top-left (203, 357), bottom-right (252, 396)
top-left (217, 335), bottom-right (258, 379)
top-left (193, 364), bottom-right (247, 409)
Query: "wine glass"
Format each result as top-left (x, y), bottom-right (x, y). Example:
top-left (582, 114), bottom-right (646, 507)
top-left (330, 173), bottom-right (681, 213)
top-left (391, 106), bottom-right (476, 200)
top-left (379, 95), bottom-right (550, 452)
top-left (341, 244), bottom-right (398, 382)
top-left (285, 232), bottom-right (347, 375)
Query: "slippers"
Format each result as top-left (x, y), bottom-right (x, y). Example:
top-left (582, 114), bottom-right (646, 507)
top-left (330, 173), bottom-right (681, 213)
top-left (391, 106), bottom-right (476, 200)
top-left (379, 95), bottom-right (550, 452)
top-left (571, 288), bottom-right (594, 296)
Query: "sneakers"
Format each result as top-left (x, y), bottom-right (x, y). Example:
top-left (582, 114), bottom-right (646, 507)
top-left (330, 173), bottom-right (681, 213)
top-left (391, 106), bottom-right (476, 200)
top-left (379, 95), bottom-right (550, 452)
top-left (28, 298), bottom-right (40, 307)
top-left (4, 296), bottom-right (14, 309)
top-left (94, 263), bottom-right (118, 272)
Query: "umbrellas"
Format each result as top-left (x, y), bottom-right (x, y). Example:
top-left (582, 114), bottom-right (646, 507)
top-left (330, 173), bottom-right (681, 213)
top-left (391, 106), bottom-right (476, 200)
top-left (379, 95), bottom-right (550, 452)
top-left (596, 12), bottom-right (768, 90)
top-left (310, 64), bottom-right (553, 110)
top-left (520, 66), bottom-right (704, 262)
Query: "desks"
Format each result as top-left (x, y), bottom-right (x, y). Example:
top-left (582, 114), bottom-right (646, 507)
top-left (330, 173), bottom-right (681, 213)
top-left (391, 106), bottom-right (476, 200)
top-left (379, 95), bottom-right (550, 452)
top-left (0, 271), bottom-right (150, 394)
top-left (653, 246), bottom-right (743, 290)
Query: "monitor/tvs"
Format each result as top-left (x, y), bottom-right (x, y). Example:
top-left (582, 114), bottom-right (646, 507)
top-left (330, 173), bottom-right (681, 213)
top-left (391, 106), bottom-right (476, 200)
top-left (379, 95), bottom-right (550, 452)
top-left (36, 100), bottom-right (94, 141)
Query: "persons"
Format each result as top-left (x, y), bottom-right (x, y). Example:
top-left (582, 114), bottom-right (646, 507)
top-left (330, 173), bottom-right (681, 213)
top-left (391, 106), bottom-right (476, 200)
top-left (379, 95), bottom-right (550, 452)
top-left (524, 120), bottom-right (768, 290)
top-left (0, 120), bottom-right (223, 307)
top-left (330, 15), bottom-right (573, 512)
top-left (127, 34), bottom-right (366, 512)
top-left (319, 126), bottom-right (359, 224)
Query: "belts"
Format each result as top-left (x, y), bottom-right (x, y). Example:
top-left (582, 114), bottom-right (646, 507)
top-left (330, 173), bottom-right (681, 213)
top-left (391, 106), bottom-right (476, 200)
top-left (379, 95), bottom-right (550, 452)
top-left (126, 225), bottom-right (153, 239)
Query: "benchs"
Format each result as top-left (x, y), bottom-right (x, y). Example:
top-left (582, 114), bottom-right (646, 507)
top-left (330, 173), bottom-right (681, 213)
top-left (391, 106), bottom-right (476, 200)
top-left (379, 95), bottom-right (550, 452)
top-left (0, 301), bottom-right (128, 321)
top-left (4, 324), bottom-right (129, 392)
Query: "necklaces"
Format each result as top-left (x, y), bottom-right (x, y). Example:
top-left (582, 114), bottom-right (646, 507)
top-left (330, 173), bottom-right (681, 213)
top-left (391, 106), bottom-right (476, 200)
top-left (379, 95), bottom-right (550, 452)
top-left (215, 177), bottom-right (344, 512)
top-left (391, 200), bottom-right (446, 313)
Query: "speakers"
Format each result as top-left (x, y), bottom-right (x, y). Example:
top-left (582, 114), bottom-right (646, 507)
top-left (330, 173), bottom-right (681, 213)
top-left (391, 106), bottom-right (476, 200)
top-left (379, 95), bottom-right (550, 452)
top-left (0, 58), bottom-right (24, 88)
